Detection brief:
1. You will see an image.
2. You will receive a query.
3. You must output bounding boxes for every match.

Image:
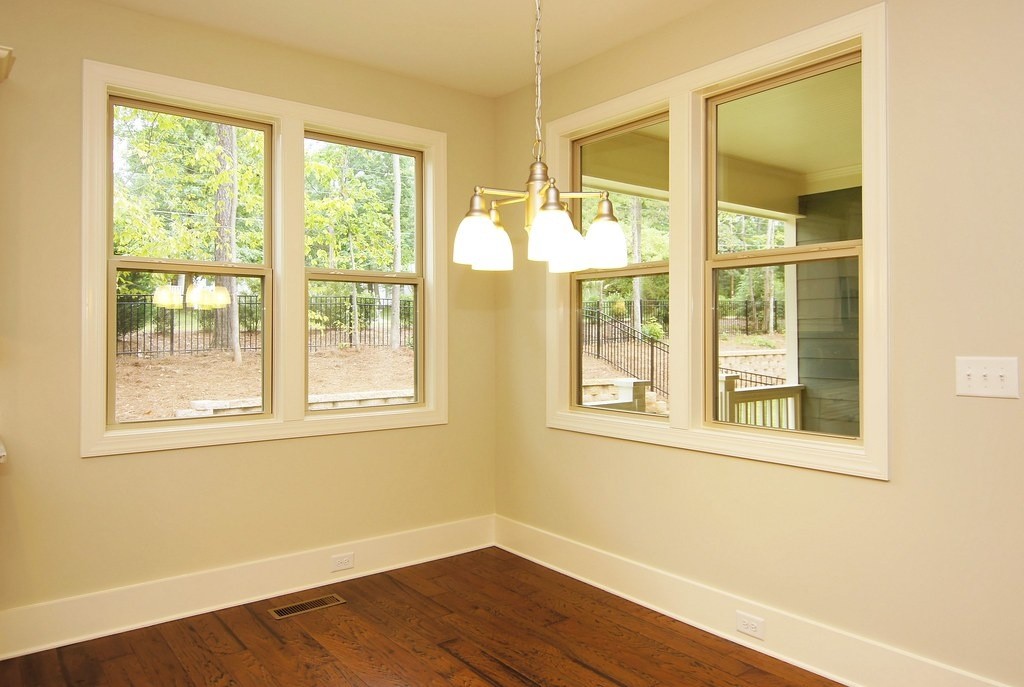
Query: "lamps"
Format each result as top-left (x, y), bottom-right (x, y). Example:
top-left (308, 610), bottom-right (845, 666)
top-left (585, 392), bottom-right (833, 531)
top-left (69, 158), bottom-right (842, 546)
top-left (151, 274), bottom-right (232, 310)
top-left (452, 0), bottom-right (629, 273)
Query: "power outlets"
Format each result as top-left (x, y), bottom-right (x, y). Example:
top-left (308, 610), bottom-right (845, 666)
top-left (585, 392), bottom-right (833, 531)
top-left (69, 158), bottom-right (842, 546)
top-left (331, 551), bottom-right (355, 573)
top-left (954, 356), bottom-right (1019, 398)
